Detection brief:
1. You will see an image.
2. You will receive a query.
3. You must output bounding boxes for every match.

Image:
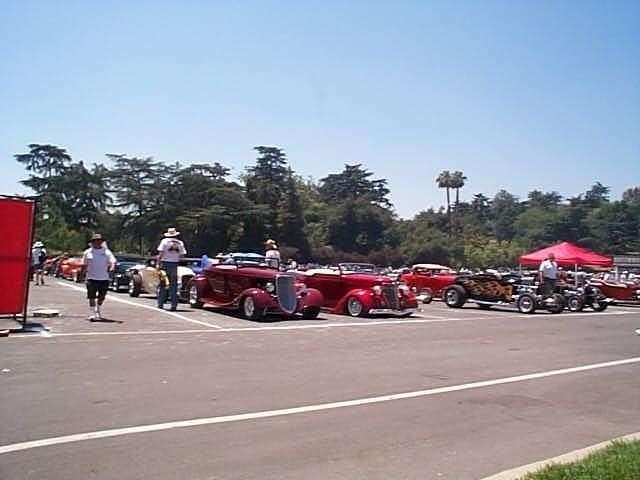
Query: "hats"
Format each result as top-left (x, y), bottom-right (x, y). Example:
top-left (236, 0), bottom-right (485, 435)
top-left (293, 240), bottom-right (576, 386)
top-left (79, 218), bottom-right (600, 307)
top-left (32, 242), bottom-right (43, 248)
top-left (164, 228), bottom-right (180, 237)
top-left (87, 234), bottom-right (104, 243)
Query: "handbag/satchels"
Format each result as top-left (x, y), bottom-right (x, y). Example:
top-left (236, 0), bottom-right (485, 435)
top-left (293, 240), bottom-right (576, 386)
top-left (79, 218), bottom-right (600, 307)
top-left (40, 252), bottom-right (48, 263)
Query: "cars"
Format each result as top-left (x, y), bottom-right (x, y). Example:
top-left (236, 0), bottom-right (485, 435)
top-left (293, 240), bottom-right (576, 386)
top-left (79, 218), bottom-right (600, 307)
top-left (223, 247), bottom-right (640, 320)
top-left (41, 249), bottom-right (221, 305)
top-left (187, 262), bottom-right (324, 321)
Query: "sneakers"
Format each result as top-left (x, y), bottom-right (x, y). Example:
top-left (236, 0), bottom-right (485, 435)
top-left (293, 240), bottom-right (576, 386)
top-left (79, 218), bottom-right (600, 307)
top-left (98, 310), bottom-right (104, 320)
top-left (90, 313), bottom-right (96, 320)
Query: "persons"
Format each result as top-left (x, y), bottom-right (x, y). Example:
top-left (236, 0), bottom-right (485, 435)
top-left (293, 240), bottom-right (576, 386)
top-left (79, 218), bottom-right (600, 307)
top-left (263, 239), bottom-right (282, 270)
top-left (32, 241), bottom-right (47, 285)
top-left (538, 253), bottom-right (558, 294)
top-left (155, 228), bottom-right (187, 311)
top-left (82, 233), bottom-right (118, 322)
